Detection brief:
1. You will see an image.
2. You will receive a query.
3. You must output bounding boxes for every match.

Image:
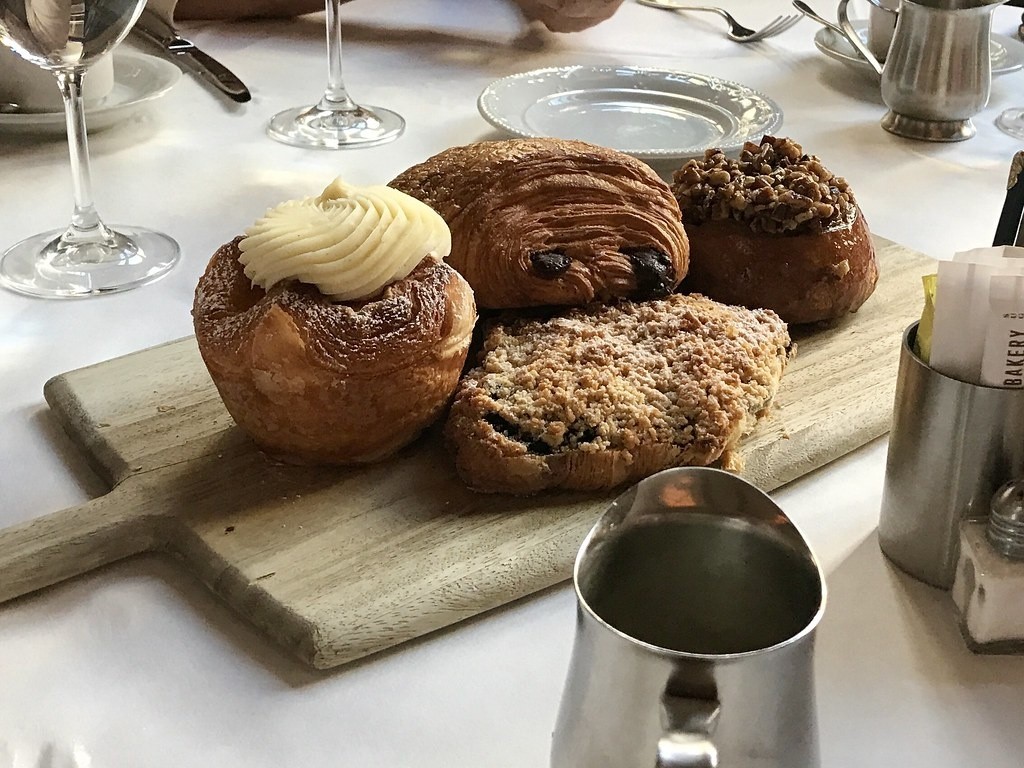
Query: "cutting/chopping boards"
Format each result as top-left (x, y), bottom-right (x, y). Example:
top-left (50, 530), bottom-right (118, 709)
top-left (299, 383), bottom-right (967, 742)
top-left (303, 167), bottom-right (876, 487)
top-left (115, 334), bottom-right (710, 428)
top-left (0, 225), bottom-right (941, 672)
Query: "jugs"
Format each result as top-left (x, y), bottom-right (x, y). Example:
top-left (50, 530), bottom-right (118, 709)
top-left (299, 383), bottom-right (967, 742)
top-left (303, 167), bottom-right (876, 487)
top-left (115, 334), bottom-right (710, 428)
top-left (550, 466), bottom-right (827, 768)
top-left (837, 0), bottom-right (1012, 143)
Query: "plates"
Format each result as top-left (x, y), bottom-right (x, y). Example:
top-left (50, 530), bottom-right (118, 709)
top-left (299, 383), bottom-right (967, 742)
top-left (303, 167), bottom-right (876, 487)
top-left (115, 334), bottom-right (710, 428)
top-left (814, 19), bottom-right (1024, 82)
top-left (0, 50), bottom-right (183, 135)
top-left (477, 65), bottom-right (784, 172)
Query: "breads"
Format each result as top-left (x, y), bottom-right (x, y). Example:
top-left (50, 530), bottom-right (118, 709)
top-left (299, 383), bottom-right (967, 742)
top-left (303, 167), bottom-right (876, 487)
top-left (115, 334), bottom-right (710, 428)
top-left (191, 134), bottom-right (879, 490)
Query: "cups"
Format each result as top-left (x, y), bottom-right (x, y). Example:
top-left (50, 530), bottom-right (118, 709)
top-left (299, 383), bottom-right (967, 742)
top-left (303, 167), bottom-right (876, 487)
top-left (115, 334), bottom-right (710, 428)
top-left (869, 0), bottom-right (905, 61)
top-left (877, 319), bottom-right (1024, 589)
top-left (0, 42), bottom-right (115, 113)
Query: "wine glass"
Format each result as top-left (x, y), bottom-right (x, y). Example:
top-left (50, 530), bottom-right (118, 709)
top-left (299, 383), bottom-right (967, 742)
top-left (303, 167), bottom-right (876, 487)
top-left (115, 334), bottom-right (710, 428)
top-left (265, 0), bottom-right (406, 151)
top-left (995, 106), bottom-right (1024, 142)
top-left (0, 0), bottom-right (182, 299)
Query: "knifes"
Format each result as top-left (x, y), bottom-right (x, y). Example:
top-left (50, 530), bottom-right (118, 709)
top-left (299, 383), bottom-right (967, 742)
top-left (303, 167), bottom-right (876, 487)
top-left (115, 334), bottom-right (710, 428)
top-left (100, 0), bottom-right (252, 102)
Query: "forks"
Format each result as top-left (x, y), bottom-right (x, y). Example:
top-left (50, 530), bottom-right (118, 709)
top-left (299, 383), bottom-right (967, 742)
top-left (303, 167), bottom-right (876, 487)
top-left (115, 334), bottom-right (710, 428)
top-left (635, 0), bottom-right (805, 43)
